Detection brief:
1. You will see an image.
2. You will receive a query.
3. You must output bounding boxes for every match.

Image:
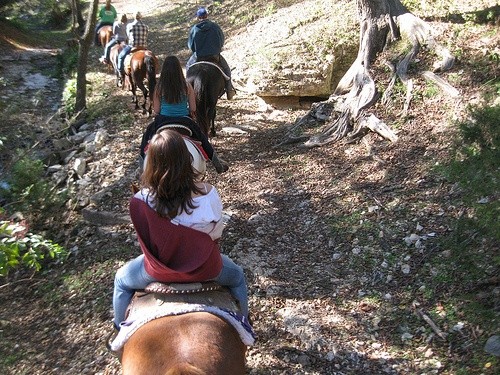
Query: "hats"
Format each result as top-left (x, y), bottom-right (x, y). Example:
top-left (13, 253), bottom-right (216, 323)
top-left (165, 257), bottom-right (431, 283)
top-left (197, 8), bottom-right (207, 16)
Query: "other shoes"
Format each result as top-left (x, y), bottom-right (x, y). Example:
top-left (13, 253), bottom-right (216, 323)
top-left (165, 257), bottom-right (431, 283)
top-left (211, 151), bottom-right (229, 174)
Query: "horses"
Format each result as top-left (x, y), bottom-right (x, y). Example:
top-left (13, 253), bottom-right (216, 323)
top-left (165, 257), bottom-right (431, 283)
top-left (96, 25), bottom-right (247, 375)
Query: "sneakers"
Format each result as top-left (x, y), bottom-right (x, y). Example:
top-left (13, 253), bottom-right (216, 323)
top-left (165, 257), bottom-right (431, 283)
top-left (105, 324), bottom-right (121, 350)
top-left (102, 57), bottom-right (109, 65)
top-left (116, 71), bottom-right (124, 80)
top-left (227, 90), bottom-right (236, 100)
